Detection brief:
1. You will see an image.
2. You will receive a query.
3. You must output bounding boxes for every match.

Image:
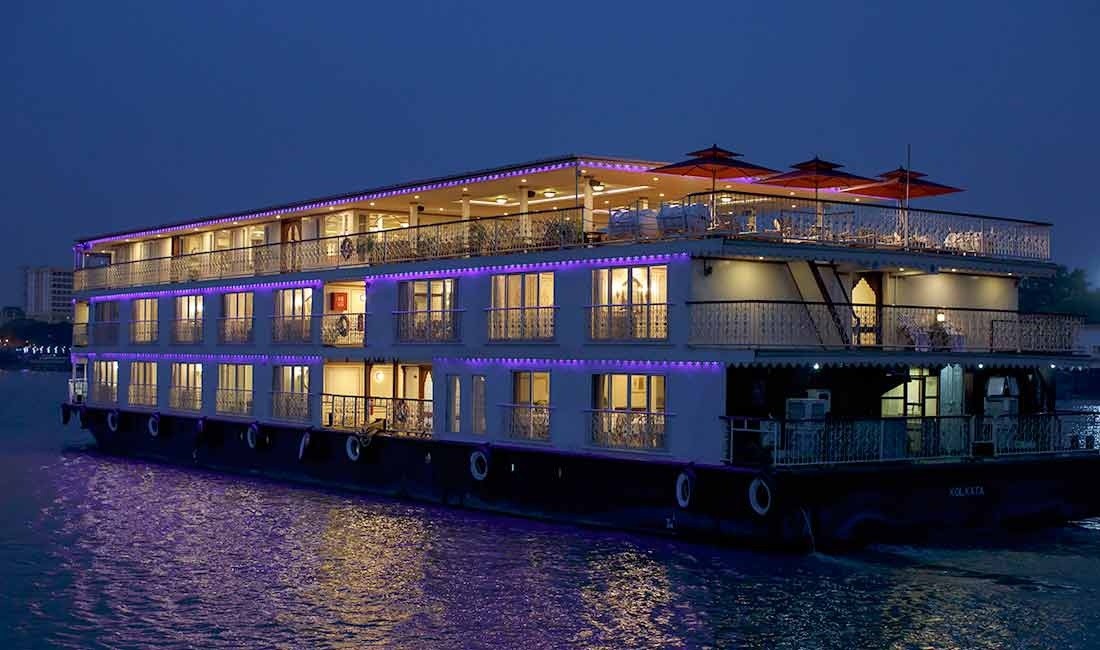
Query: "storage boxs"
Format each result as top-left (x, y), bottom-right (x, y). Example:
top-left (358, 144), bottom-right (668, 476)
top-left (983, 396), bottom-right (1019, 416)
top-left (785, 397), bottom-right (826, 422)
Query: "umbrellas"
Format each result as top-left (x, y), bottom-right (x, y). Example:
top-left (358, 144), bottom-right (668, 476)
top-left (750, 155), bottom-right (884, 240)
top-left (644, 144), bottom-right (781, 228)
top-left (838, 166), bottom-right (969, 238)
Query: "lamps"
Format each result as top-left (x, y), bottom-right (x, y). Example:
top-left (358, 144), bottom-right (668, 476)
top-left (544, 192), bottom-right (556, 198)
top-left (592, 185), bottom-right (605, 192)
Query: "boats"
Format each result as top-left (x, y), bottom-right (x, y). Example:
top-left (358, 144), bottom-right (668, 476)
top-left (61, 140), bottom-right (1100, 547)
